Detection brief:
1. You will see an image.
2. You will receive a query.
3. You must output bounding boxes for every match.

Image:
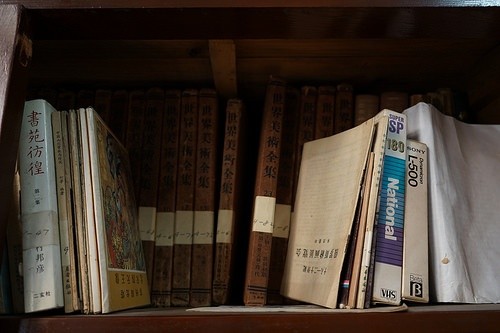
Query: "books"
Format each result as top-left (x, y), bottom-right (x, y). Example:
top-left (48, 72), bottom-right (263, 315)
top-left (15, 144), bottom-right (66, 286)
top-left (7, 80), bottom-right (457, 315)
